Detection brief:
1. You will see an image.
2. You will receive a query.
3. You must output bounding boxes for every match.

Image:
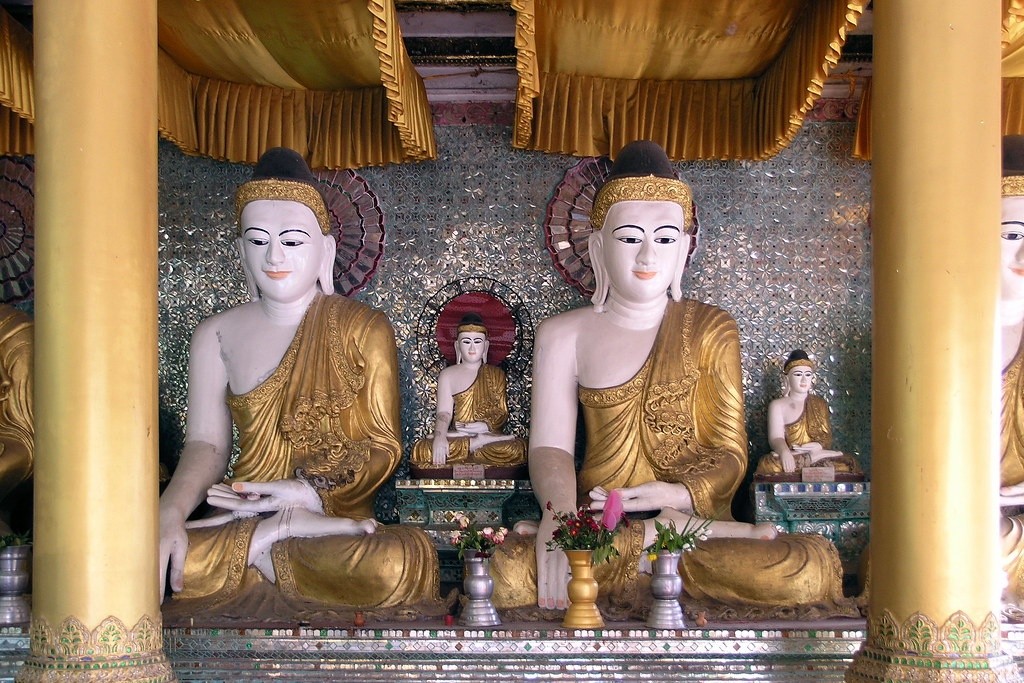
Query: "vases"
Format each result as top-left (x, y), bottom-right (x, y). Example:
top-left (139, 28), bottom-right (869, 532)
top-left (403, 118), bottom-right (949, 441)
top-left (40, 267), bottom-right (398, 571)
top-left (459, 549), bottom-right (502, 626)
top-left (563, 550), bottom-right (606, 628)
top-left (643, 548), bottom-right (684, 629)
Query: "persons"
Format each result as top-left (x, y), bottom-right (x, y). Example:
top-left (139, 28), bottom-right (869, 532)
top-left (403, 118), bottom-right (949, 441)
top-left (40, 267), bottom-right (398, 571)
top-left (485, 140), bottom-right (843, 607)
top-left (1001, 135), bottom-right (1024, 645)
top-left (757, 349), bottom-right (862, 475)
top-left (410, 311), bottom-right (527, 469)
top-left (160, 147), bottom-right (437, 610)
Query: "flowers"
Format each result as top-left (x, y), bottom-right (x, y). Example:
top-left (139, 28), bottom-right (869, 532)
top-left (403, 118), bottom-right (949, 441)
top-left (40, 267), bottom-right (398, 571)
top-left (450, 514), bottom-right (509, 562)
top-left (640, 504), bottom-right (730, 561)
top-left (545, 500), bottom-right (631, 567)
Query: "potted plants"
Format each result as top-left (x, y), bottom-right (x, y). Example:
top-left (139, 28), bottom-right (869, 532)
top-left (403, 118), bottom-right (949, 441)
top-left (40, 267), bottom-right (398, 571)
top-left (0, 533), bottom-right (32, 629)
